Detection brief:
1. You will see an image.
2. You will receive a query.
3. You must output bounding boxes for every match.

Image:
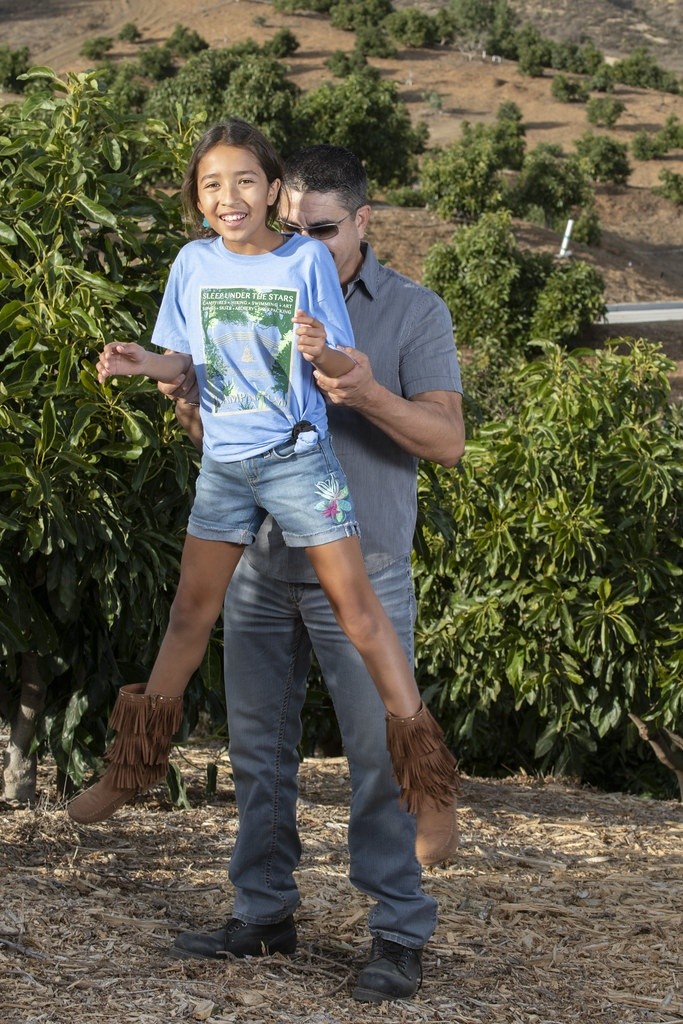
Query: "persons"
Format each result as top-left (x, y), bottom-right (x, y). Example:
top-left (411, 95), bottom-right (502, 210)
top-left (72, 119), bottom-right (461, 868)
top-left (149, 141), bottom-right (463, 1003)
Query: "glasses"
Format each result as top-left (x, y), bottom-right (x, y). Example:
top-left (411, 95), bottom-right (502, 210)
top-left (275, 210), bottom-right (354, 240)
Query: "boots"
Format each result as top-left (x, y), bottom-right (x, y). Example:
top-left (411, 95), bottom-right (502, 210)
top-left (384, 700), bottom-right (460, 865)
top-left (67, 682), bottom-right (185, 823)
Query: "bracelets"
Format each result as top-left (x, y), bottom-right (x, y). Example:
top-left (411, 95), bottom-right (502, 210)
top-left (188, 399), bottom-right (200, 408)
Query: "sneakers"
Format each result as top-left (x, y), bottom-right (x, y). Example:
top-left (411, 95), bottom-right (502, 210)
top-left (172, 920), bottom-right (297, 961)
top-left (353, 937), bottom-right (422, 1002)
